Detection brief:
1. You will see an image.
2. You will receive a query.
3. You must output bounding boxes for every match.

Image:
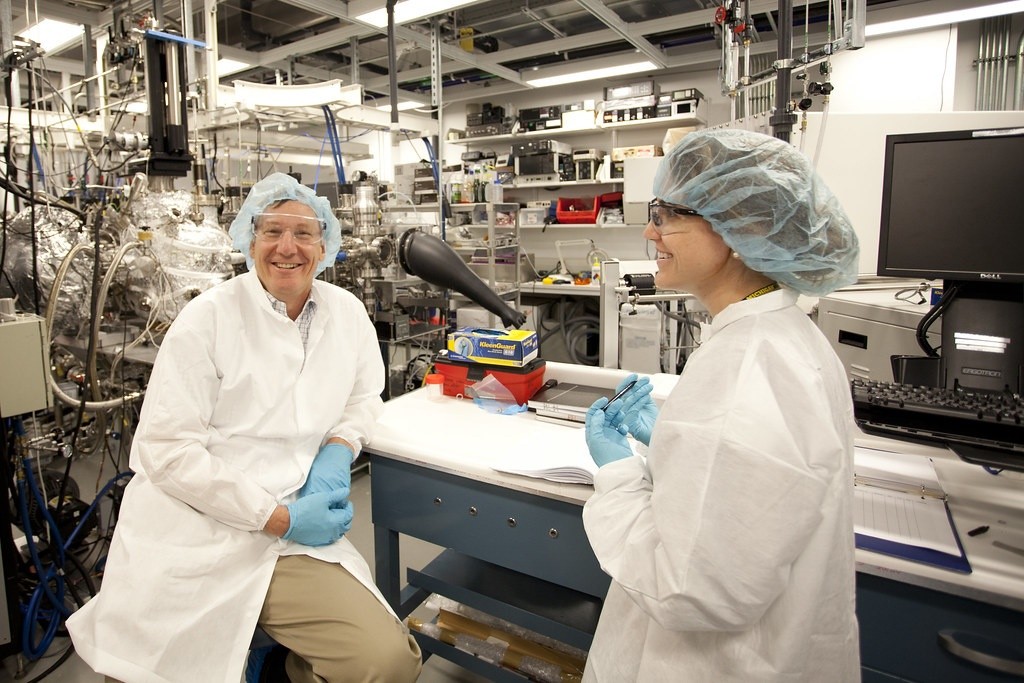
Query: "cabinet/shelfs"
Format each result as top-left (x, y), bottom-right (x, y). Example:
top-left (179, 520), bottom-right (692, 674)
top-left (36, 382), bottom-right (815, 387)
top-left (443, 113), bottom-right (710, 228)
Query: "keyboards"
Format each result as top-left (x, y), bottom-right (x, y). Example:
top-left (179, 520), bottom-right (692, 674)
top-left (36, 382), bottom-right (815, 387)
top-left (849, 380), bottom-right (1024, 447)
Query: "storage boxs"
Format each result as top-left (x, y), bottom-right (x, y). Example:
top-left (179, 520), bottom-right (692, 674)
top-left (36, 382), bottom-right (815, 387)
top-left (449, 323), bottom-right (542, 367)
top-left (602, 95), bottom-right (659, 111)
top-left (673, 87), bottom-right (705, 102)
top-left (658, 93), bottom-right (673, 105)
top-left (603, 103), bottom-right (658, 124)
top-left (611, 145), bottom-right (662, 161)
top-left (434, 352), bottom-right (550, 398)
top-left (520, 207), bottom-right (550, 225)
top-left (602, 80), bottom-right (661, 101)
top-left (656, 104), bottom-right (671, 117)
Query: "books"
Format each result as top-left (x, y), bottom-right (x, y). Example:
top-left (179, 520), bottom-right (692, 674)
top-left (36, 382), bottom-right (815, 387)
top-left (855, 446), bottom-right (973, 574)
top-left (528, 381), bottom-right (616, 424)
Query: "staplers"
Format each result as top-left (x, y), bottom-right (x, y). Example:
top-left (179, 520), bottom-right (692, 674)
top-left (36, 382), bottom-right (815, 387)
top-left (528, 378), bottom-right (558, 411)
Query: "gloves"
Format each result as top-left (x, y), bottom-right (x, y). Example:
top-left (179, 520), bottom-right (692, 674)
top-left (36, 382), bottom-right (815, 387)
top-left (616, 374), bottom-right (660, 446)
top-left (280, 487), bottom-right (354, 547)
top-left (584, 397), bottom-right (634, 468)
top-left (299, 444), bottom-right (352, 509)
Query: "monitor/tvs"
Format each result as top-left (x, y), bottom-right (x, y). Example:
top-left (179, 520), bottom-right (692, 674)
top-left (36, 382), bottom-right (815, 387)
top-left (877, 126), bottom-right (1024, 286)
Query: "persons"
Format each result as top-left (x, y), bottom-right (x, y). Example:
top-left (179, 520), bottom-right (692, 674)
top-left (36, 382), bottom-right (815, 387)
top-left (583, 128), bottom-right (862, 683)
top-left (64, 172), bottom-right (422, 683)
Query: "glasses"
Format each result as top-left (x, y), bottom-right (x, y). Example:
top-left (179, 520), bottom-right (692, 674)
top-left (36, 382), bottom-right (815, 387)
top-left (647, 198), bottom-right (727, 228)
top-left (251, 213), bottom-right (327, 244)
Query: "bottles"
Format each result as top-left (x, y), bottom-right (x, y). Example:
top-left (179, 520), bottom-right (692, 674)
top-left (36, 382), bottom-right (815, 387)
top-left (461, 163), bottom-right (504, 204)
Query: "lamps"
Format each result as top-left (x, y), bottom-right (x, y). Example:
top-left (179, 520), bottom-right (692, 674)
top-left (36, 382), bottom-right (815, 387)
top-left (365, 96), bottom-right (425, 113)
top-left (519, 44), bottom-right (666, 87)
top-left (345, 0), bottom-right (488, 32)
top-left (11, 10), bottom-right (88, 59)
top-left (217, 54), bottom-right (250, 76)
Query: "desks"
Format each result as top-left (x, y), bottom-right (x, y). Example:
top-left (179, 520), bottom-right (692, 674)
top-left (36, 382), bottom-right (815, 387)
top-left (349, 351), bottom-right (1024, 683)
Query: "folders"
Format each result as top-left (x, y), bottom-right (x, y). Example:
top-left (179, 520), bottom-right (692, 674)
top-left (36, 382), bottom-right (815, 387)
top-left (854, 445), bottom-right (972, 575)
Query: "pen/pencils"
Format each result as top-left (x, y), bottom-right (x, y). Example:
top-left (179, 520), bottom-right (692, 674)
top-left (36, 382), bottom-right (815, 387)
top-left (600, 380), bottom-right (637, 413)
